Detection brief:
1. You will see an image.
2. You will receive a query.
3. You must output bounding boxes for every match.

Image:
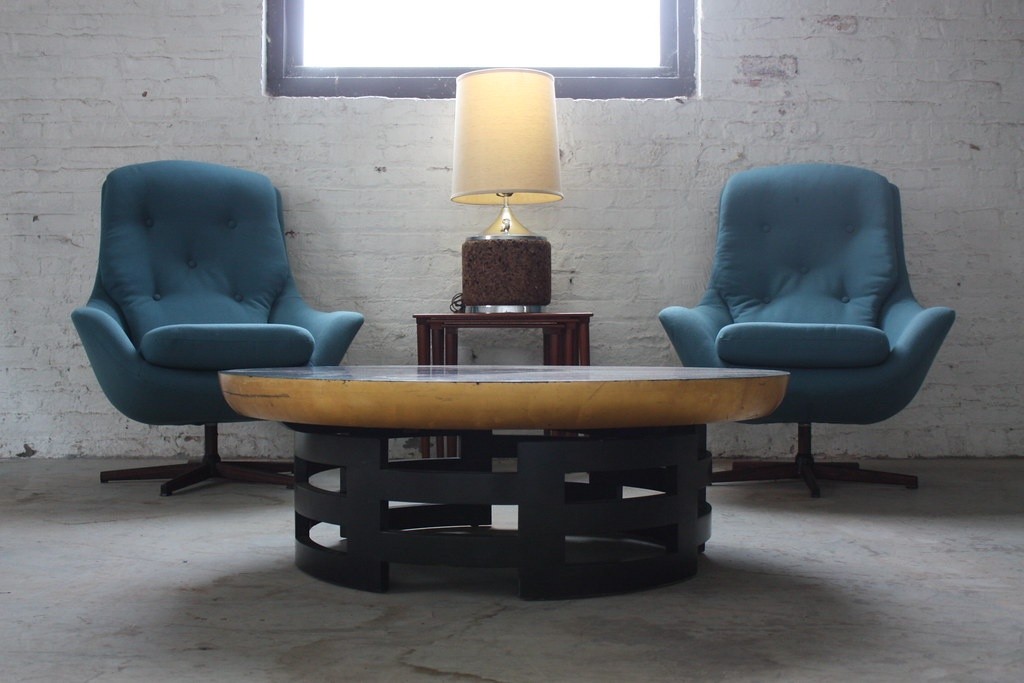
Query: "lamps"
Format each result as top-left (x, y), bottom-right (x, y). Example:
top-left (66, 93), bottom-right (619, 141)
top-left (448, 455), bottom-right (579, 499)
top-left (448, 68), bottom-right (563, 312)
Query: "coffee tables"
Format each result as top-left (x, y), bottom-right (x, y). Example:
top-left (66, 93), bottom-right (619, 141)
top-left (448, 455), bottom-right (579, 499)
top-left (220, 365), bottom-right (792, 600)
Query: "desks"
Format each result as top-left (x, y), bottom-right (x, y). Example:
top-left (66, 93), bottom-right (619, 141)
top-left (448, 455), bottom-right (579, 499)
top-left (413, 312), bottom-right (594, 458)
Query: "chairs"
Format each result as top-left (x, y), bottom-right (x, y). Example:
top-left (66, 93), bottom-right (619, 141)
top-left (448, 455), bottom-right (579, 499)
top-left (72, 160), bottom-right (364, 496)
top-left (658, 163), bottom-right (957, 497)
